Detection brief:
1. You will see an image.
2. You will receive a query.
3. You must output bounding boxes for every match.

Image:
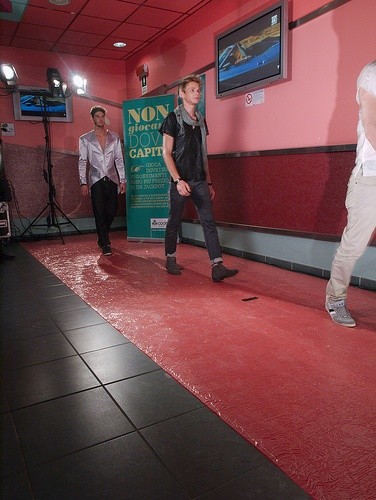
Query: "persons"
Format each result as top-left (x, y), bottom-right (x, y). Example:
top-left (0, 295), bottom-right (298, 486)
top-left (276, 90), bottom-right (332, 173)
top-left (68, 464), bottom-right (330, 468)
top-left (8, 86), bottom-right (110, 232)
top-left (324, 58), bottom-right (376, 328)
top-left (79, 106), bottom-right (127, 255)
top-left (158, 76), bottom-right (238, 281)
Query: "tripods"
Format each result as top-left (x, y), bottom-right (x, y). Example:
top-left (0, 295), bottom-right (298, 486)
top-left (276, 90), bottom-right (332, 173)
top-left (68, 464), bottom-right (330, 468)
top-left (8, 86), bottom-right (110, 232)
top-left (19, 95), bottom-right (82, 244)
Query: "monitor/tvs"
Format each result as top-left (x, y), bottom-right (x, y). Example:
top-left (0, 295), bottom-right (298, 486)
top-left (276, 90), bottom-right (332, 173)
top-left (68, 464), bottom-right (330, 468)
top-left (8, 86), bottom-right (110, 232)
top-left (12, 86), bottom-right (76, 123)
top-left (215, 0), bottom-right (289, 100)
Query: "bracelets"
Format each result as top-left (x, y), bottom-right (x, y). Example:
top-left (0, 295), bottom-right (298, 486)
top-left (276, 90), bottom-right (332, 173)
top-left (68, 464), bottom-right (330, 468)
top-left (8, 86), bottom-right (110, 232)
top-left (208, 183), bottom-right (213, 185)
top-left (174, 178), bottom-right (183, 185)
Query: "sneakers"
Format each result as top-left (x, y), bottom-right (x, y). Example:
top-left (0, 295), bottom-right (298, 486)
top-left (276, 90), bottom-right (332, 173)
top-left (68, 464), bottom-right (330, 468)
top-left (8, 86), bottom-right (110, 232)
top-left (102, 245), bottom-right (112, 255)
top-left (166, 257), bottom-right (181, 274)
top-left (212, 264), bottom-right (239, 281)
top-left (325, 300), bottom-right (357, 327)
